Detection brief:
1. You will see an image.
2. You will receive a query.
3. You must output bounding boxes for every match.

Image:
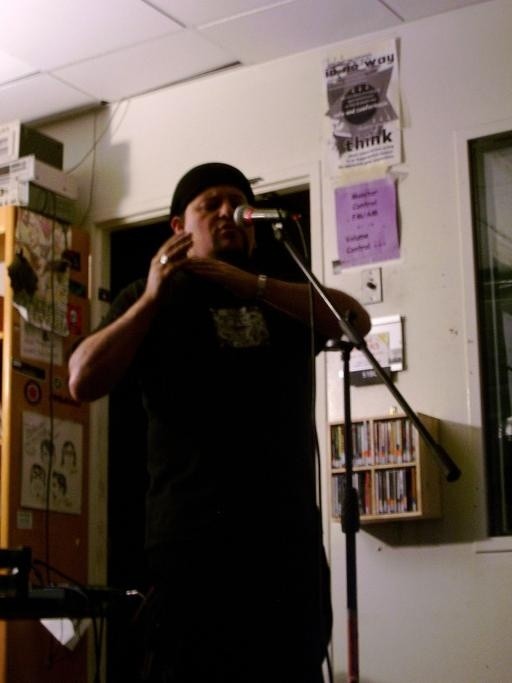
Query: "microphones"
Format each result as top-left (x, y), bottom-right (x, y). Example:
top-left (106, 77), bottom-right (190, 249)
top-left (233, 205), bottom-right (286, 226)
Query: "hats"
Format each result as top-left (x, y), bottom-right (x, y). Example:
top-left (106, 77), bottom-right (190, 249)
top-left (169, 161), bottom-right (258, 221)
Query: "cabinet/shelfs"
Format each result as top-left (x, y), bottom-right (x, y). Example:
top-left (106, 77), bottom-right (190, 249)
top-left (327, 411), bottom-right (442, 525)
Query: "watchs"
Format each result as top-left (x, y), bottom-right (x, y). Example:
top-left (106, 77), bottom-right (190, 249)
top-left (256, 273), bottom-right (267, 307)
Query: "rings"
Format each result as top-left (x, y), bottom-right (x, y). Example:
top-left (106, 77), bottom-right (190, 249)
top-left (158, 254), bottom-right (170, 265)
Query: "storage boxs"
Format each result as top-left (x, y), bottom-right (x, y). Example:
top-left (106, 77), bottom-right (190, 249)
top-left (0, 119), bottom-right (80, 224)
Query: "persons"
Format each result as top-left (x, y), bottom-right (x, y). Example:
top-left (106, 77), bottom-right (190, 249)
top-left (26, 440), bottom-right (77, 508)
top-left (64, 161), bottom-right (373, 683)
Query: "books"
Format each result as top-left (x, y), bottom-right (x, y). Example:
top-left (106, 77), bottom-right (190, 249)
top-left (330, 418), bottom-right (419, 517)
